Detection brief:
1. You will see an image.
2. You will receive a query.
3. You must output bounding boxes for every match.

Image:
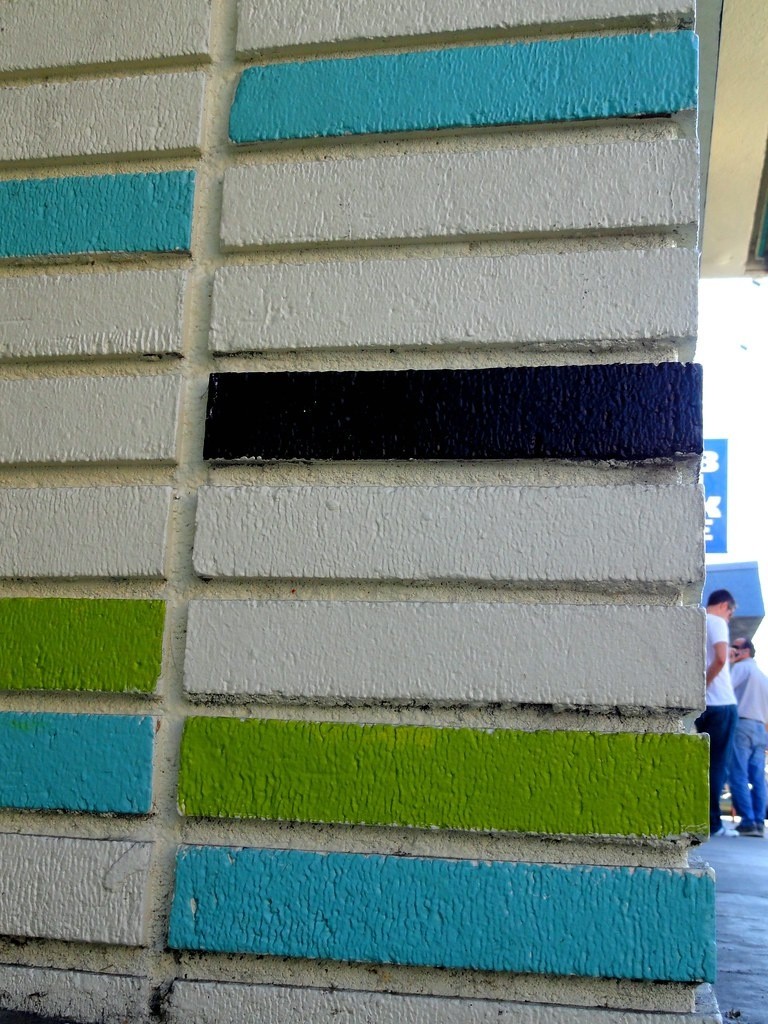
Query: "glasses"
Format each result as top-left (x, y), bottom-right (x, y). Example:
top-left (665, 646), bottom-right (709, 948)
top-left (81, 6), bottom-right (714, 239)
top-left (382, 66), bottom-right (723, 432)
top-left (732, 645), bottom-right (746, 650)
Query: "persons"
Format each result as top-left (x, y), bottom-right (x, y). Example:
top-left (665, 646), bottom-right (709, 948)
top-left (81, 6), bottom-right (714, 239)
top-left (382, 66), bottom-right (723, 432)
top-left (695, 589), bottom-right (741, 838)
top-left (730, 636), bottom-right (768, 837)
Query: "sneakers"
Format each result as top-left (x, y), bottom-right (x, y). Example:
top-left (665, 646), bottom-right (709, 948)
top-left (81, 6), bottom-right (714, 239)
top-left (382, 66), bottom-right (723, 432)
top-left (734, 819), bottom-right (764, 838)
